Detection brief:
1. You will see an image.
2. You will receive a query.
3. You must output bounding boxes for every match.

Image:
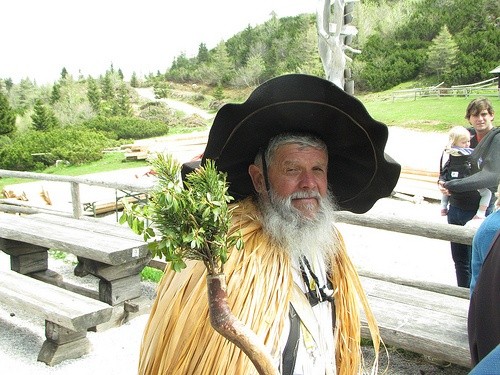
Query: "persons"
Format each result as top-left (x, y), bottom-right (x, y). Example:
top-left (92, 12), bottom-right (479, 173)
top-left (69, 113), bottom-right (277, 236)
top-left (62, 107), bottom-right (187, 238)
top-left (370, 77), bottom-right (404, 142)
top-left (436, 97), bottom-right (500, 289)
top-left (138, 74), bottom-right (402, 375)
top-left (463, 208), bottom-right (500, 375)
top-left (439, 125), bottom-right (492, 220)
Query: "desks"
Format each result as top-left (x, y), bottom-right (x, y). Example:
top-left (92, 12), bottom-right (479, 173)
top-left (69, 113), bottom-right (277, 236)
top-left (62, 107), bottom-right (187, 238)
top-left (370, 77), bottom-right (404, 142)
top-left (0, 212), bottom-right (162, 334)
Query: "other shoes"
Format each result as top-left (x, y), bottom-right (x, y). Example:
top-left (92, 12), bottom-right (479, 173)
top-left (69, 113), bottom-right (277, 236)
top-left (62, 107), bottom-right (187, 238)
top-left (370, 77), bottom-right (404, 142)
top-left (471, 215), bottom-right (485, 221)
top-left (440, 208), bottom-right (449, 215)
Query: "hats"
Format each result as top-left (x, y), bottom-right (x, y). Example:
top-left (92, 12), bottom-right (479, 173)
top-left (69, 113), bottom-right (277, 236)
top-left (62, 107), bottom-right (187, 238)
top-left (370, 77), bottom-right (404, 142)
top-left (180, 73), bottom-right (401, 215)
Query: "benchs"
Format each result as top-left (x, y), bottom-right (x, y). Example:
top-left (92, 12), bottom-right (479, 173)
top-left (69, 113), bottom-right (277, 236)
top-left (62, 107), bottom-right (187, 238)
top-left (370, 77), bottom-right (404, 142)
top-left (0, 265), bottom-right (112, 367)
top-left (357, 274), bottom-right (472, 370)
